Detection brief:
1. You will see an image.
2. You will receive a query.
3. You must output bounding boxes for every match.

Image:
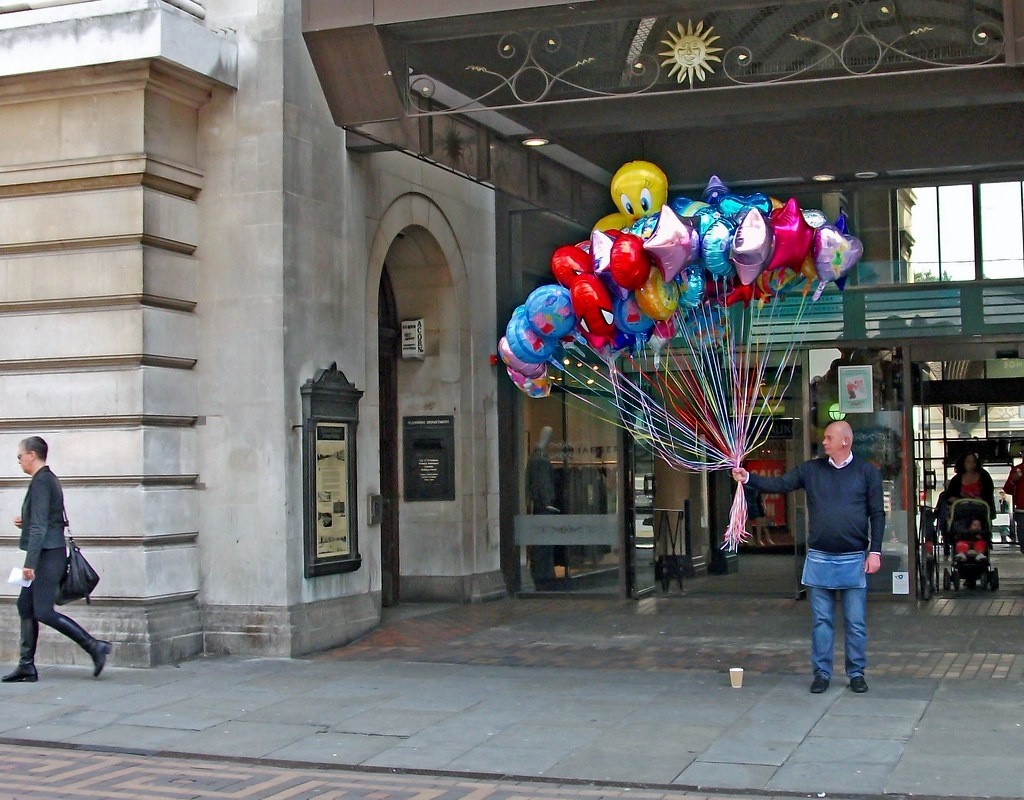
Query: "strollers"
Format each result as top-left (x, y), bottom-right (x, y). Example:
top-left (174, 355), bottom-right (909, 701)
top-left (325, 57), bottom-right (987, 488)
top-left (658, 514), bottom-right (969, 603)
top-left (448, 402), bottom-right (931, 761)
top-left (943, 498), bottom-right (999, 592)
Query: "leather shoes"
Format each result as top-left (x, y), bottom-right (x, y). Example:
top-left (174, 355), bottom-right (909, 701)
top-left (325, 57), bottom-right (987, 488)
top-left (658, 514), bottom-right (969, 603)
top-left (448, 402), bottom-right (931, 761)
top-left (809, 675), bottom-right (830, 693)
top-left (849, 676), bottom-right (869, 692)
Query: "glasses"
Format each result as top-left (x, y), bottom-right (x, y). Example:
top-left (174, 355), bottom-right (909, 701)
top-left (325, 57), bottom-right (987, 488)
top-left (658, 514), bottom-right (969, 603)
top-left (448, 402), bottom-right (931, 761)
top-left (15, 452), bottom-right (32, 459)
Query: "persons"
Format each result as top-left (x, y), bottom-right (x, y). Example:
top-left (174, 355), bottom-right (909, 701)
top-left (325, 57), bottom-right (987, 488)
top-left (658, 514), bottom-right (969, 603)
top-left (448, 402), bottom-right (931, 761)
top-left (744, 470), bottom-right (775, 546)
top-left (996, 439), bottom-right (1024, 555)
top-left (526, 425), bottom-right (560, 592)
top-left (732, 420), bottom-right (887, 693)
top-left (2, 436), bottom-right (113, 683)
top-left (933, 451), bottom-right (996, 591)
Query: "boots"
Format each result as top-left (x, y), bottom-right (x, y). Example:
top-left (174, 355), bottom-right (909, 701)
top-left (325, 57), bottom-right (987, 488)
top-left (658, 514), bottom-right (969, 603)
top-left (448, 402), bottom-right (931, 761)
top-left (41, 611), bottom-right (112, 677)
top-left (1, 619), bottom-right (39, 683)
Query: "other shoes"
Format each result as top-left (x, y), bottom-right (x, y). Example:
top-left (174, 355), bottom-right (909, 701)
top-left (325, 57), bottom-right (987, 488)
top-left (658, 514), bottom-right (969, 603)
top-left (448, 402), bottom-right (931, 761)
top-left (954, 553), bottom-right (967, 562)
top-left (975, 553), bottom-right (987, 562)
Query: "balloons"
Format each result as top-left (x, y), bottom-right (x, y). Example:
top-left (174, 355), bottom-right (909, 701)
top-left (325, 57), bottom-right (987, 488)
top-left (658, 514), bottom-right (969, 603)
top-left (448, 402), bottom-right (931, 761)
top-left (499, 160), bottom-right (863, 398)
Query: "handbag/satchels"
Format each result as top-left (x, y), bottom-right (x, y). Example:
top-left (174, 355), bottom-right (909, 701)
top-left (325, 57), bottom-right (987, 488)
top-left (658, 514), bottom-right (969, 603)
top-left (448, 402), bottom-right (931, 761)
top-left (54, 504), bottom-right (99, 607)
top-left (653, 510), bottom-right (696, 581)
